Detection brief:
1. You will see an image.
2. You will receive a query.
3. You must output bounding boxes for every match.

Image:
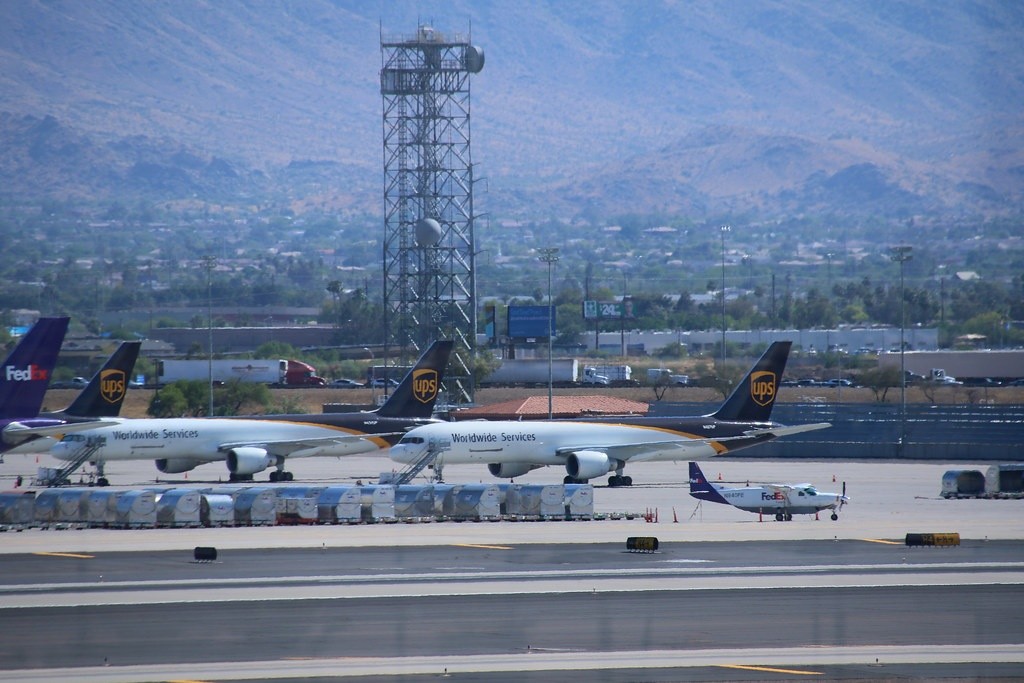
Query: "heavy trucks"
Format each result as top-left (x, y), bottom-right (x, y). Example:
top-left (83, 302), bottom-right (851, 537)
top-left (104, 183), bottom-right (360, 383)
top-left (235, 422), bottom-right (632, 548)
top-left (156, 357), bottom-right (326, 389)
top-left (476, 356), bottom-right (610, 389)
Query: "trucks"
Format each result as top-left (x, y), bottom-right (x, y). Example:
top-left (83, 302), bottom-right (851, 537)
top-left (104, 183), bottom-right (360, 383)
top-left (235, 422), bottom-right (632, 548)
top-left (644, 368), bottom-right (689, 388)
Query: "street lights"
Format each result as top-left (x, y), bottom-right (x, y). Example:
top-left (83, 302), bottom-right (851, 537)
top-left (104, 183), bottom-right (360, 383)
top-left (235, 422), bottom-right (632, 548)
top-left (364, 347), bottom-right (376, 405)
top-left (718, 225), bottom-right (733, 379)
top-left (889, 244), bottom-right (916, 459)
top-left (538, 247), bottom-right (560, 420)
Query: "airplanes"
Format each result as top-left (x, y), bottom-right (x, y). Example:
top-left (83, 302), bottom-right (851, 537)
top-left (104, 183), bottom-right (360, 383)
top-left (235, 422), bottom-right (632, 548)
top-left (0, 315), bottom-right (144, 468)
top-left (687, 459), bottom-right (851, 520)
top-left (38, 335), bottom-right (459, 483)
top-left (385, 339), bottom-right (836, 489)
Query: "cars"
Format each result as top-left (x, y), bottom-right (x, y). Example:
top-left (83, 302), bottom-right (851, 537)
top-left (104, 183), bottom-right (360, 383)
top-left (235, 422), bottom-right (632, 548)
top-left (71, 376), bottom-right (90, 389)
top-left (777, 346), bottom-right (1024, 387)
top-left (367, 376), bottom-right (399, 387)
top-left (327, 378), bottom-right (365, 390)
top-left (129, 379), bottom-right (144, 389)
top-left (51, 381), bottom-right (65, 390)
